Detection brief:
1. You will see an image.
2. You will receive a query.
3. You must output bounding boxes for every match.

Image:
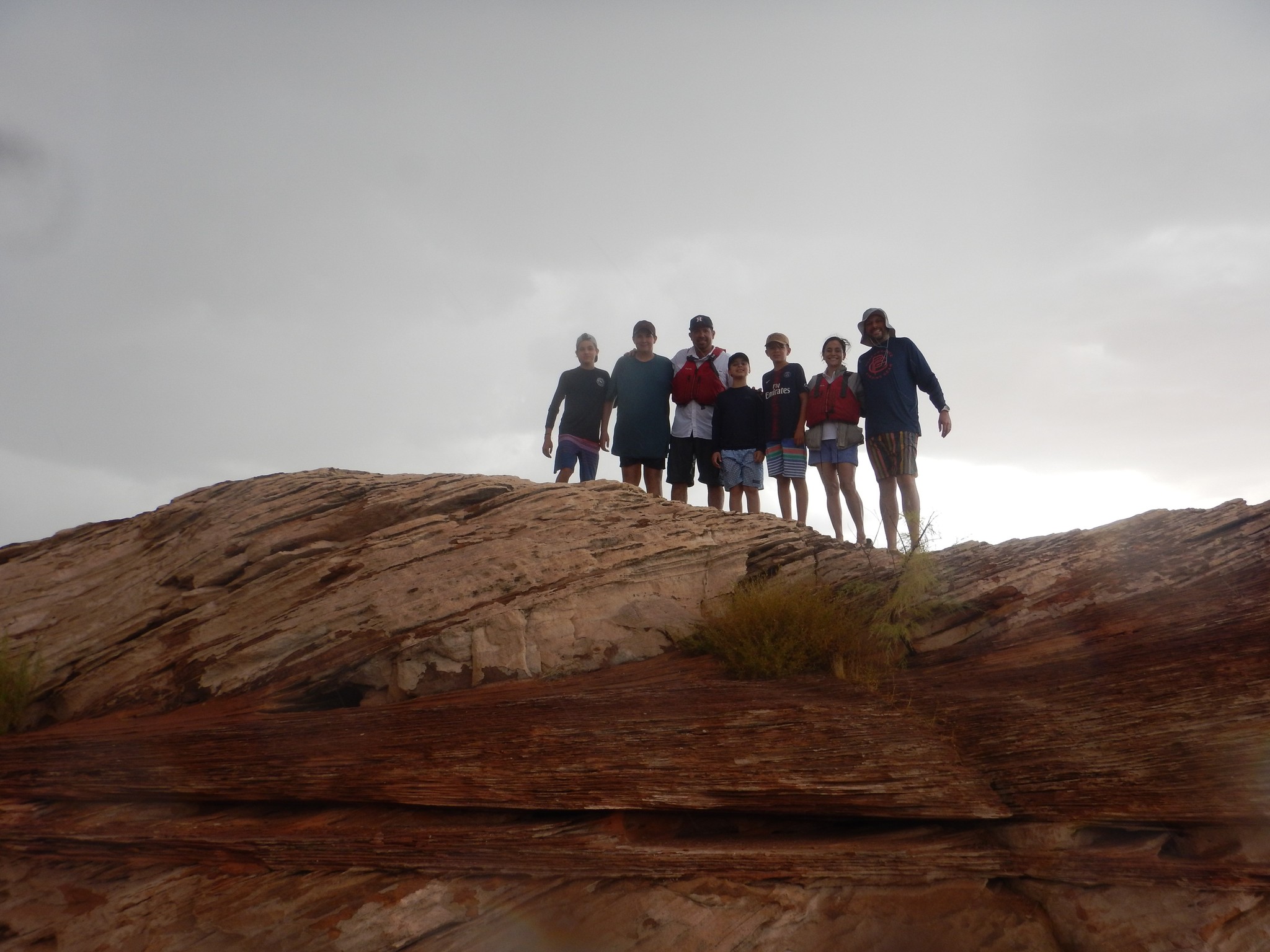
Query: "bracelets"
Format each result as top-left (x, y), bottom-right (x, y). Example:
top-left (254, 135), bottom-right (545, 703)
top-left (545, 433), bottom-right (551, 436)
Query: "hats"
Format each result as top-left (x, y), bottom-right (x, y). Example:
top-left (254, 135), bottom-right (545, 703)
top-left (765, 333), bottom-right (789, 347)
top-left (689, 315), bottom-right (713, 330)
top-left (576, 333), bottom-right (598, 363)
top-left (633, 320), bottom-right (656, 339)
top-left (858, 308), bottom-right (895, 347)
top-left (728, 352), bottom-right (749, 368)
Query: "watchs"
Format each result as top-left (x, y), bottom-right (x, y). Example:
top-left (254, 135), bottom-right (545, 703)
top-left (941, 405), bottom-right (950, 411)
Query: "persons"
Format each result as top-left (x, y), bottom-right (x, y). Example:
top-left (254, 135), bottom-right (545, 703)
top-left (762, 333), bottom-right (808, 523)
top-left (857, 307), bottom-right (952, 553)
top-left (807, 337), bottom-right (867, 545)
top-left (624, 315), bottom-right (763, 511)
top-left (542, 333), bottom-right (617, 483)
top-left (599, 321), bottom-right (673, 498)
top-left (712, 352), bottom-right (764, 513)
